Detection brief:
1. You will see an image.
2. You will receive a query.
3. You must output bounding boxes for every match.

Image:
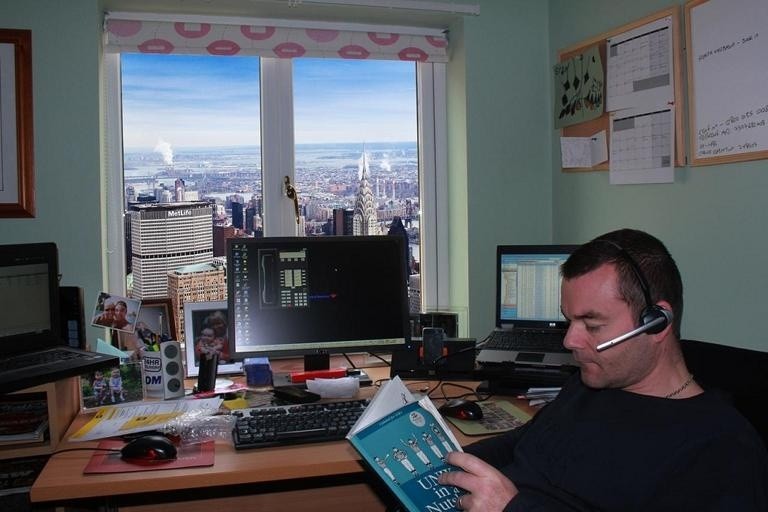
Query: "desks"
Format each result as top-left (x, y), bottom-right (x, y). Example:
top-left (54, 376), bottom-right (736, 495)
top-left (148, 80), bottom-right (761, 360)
top-left (29, 352), bottom-right (547, 512)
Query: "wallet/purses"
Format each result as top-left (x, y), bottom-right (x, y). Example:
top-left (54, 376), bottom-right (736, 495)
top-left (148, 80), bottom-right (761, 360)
top-left (273, 385), bottom-right (321, 404)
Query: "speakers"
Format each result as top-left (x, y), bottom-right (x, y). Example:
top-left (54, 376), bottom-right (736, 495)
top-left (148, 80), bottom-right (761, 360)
top-left (159, 341), bottom-right (186, 400)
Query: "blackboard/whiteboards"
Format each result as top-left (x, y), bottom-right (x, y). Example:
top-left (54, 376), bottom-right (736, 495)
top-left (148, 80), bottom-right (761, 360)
top-left (684, 0), bottom-right (768, 167)
top-left (557, 4), bottom-right (686, 173)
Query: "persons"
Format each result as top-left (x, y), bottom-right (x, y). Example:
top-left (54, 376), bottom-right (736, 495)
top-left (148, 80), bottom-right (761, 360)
top-left (92, 366), bottom-right (128, 404)
top-left (438, 229), bottom-right (768, 512)
top-left (374, 425), bottom-right (453, 486)
top-left (197, 311), bottom-right (231, 364)
top-left (95, 297), bottom-right (133, 329)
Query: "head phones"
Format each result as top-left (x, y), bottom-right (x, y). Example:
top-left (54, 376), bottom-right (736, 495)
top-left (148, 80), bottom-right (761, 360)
top-left (590, 237), bottom-right (673, 336)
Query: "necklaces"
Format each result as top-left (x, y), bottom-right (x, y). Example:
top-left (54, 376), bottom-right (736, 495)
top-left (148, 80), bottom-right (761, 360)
top-left (656, 369), bottom-right (697, 401)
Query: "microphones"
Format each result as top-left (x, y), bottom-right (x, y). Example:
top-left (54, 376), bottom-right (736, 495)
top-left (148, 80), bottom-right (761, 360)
top-left (597, 317), bottom-right (666, 352)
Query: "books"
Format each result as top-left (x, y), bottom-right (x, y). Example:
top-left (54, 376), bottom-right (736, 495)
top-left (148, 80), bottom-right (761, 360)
top-left (0, 392), bottom-right (49, 446)
top-left (346, 377), bottom-right (472, 512)
top-left (2, 459), bottom-right (46, 496)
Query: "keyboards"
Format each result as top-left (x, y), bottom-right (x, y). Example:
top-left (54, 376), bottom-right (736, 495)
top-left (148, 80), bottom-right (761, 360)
top-left (230, 397), bottom-right (375, 449)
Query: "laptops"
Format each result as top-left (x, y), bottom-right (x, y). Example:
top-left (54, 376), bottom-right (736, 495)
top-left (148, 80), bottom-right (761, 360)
top-left (475, 243), bottom-right (583, 370)
top-left (1, 242), bottom-right (120, 393)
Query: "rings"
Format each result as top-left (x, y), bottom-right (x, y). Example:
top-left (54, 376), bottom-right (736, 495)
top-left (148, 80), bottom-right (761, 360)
top-left (456, 492), bottom-right (464, 507)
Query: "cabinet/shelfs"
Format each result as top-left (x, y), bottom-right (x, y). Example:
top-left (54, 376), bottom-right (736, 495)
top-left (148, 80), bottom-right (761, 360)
top-left (0, 376), bottom-right (83, 512)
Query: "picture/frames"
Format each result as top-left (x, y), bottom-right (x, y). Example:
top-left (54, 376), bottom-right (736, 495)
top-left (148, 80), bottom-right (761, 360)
top-left (183, 301), bottom-right (265, 377)
top-left (0, 27), bottom-right (36, 218)
top-left (79, 361), bottom-right (146, 414)
top-left (112, 297), bottom-right (178, 368)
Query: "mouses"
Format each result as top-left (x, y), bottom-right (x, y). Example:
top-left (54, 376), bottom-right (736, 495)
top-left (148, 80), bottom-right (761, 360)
top-left (439, 399), bottom-right (484, 420)
top-left (120, 434), bottom-right (177, 460)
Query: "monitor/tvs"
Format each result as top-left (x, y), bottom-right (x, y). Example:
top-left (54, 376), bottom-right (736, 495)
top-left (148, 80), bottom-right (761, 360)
top-left (225, 234), bottom-right (412, 390)
